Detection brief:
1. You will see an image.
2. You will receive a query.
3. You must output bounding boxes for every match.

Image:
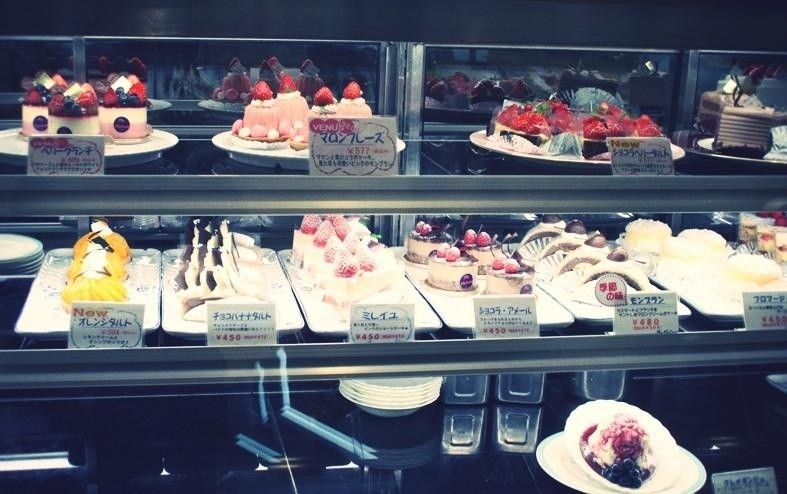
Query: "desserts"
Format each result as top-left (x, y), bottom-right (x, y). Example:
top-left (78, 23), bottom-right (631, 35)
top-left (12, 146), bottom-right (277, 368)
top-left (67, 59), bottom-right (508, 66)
top-left (403, 221), bottom-right (536, 297)
top-left (518, 213), bottom-right (787, 309)
top-left (291, 214), bottom-right (406, 309)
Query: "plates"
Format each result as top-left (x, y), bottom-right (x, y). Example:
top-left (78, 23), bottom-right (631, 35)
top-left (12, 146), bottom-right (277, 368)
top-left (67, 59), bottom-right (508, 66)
top-left (276, 246), bottom-right (445, 343)
top-left (387, 246), bottom-right (576, 337)
top-left (536, 428), bottom-right (708, 493)
top-left (0, 232), bottom-right (44, 274)
top-left (768, 373), bottom-right (787, 398)
top-left (12, 246), bottom-right (161, 344)
top-left (426, 95), bottom-right (500, 115)
top-left (210, 130), bottom-right (407, 171)
top-left (162, 247), bottom-right (307, 344)
top-left (117, 97), bottom-right (173, 121)
top-left (468, 129), bottom-right (687, 166)
top-left (339, 377), bottom-right (445, 419)
top-left (698, 136), bottom-right (787, 167)
top-left (513, 246), bottom-right (692, 330)
top-left (610, 240), bottom-right (784, 324)
top-left (197, 97), bottom-right (339, 119)
top-left (342, 411), bottom-right (440, 472)
top-left (0, 127), bottom-right (182, 166)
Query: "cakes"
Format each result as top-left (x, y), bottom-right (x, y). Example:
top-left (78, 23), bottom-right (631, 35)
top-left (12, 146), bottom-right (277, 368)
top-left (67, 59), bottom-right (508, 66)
top-left (712, 67), bottom-right (787, 159)
top-left (423, 71), bottom-right (535, 114)
top-left (214, 55), bottom-right (325, 102)
top-left (231, 75), bottom-right (372, 149)
top-left (16, 71), bottom-right (150, 140)
top-left (486, 98), bottom-right (662, 161)
top-left (694, 70), bottom-right (724, 139)
top-left (61, 220), bottom-right (132, 314)
top-left (173, 219), bottom-right (267, 322)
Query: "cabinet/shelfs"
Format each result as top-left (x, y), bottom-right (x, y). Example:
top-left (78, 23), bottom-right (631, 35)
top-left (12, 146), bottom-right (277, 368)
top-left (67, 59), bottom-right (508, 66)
top-left (0, 0), bottom-right (786, 493)
